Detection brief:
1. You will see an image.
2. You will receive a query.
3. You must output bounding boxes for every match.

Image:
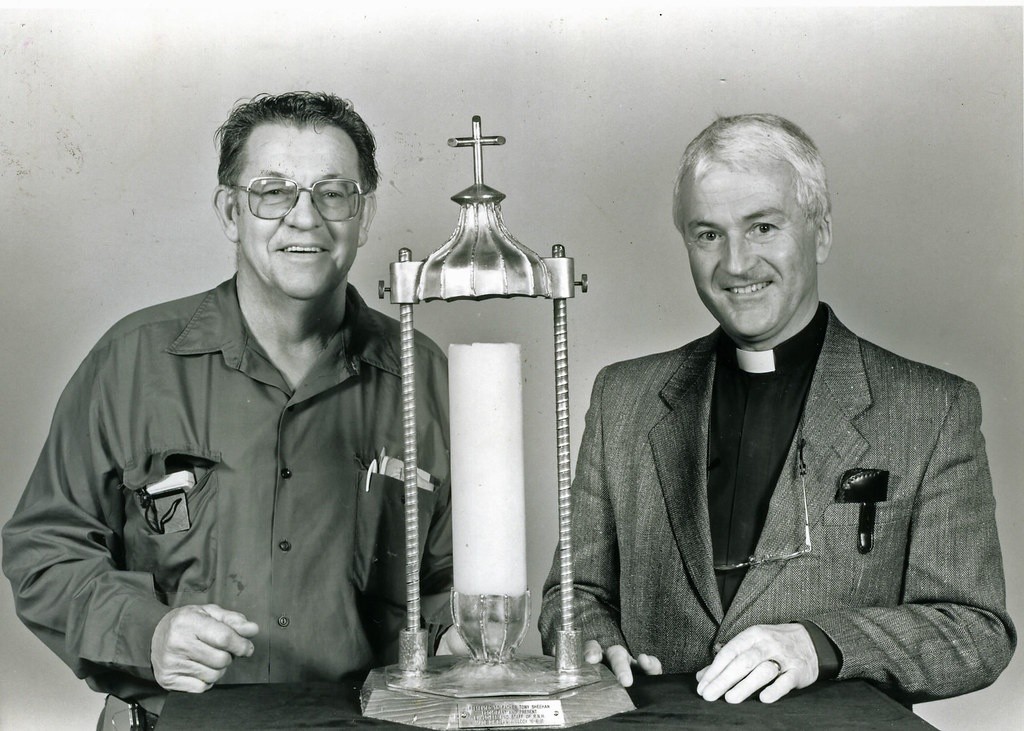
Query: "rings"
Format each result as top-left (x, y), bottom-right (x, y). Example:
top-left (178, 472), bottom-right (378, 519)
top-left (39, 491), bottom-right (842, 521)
top-left (768, 659), bottom-right (782, 676)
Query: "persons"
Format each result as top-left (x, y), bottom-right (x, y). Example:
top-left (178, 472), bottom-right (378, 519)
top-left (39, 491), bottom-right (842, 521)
top-left (0, 88), bottom-right (486, 729)
top-left (536, 114), bottom-right (1018, 713)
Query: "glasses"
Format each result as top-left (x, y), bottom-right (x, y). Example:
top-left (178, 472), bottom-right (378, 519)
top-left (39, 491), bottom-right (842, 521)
top-left (712, 438), bottom-right (814, 572)
top-left (227, 175), bottom-right (369, 223)
